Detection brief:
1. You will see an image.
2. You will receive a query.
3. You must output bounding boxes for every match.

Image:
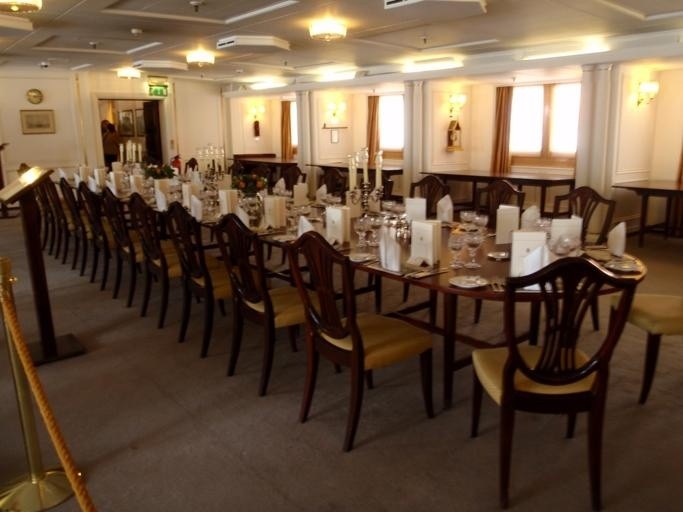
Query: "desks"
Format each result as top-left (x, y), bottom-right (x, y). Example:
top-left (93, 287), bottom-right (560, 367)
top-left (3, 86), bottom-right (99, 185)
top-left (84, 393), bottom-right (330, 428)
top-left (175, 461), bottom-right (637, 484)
top-left (613, 176), bottom-right (681, 246)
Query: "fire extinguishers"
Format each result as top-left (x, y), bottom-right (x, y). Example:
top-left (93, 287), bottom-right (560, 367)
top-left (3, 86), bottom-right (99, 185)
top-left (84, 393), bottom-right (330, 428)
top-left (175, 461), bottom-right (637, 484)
top-left (170, 155), bottom-right (182, 176)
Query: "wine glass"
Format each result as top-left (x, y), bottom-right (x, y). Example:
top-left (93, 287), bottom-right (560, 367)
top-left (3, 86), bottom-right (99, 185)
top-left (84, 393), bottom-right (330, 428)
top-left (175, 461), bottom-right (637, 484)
top-left (102, 175), bottom-right (313, 233)
top-left (353, 201), bottom-right (581, 270)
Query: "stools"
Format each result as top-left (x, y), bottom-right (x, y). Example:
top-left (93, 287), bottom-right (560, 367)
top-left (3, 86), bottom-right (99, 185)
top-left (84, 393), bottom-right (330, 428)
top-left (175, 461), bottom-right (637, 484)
top-left (607, 277), bottom-right (681, 408)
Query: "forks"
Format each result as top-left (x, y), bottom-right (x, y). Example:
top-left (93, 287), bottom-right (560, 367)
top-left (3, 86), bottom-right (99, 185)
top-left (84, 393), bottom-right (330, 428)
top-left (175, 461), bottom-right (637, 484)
top-left (488, 275), bottom-right (504, 294)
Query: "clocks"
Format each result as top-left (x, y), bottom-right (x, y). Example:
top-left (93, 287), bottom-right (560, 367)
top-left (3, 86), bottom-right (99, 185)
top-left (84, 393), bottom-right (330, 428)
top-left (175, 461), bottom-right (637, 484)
top-left (26, 87), bottom-right (43, 105)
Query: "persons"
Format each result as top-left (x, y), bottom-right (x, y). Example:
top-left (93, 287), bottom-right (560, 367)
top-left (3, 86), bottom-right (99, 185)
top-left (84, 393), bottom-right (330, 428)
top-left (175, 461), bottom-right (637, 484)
top-left (103, 123), bottom-right (119, 166)
top-left (102, 120), bottom-right (110, 134)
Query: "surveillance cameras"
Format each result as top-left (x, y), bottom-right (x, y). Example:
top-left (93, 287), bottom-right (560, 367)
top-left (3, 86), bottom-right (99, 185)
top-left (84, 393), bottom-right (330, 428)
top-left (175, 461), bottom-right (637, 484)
top-left (40, 62), bottom-right (48, 69)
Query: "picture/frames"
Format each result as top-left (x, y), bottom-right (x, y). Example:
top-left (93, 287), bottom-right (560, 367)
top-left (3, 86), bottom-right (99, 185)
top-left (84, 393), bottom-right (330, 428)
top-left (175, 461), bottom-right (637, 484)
top-left (117, 110), bottom-right (134, 137)
top-left (135, 108), bottom-right (145, 138)
top-left (20, 108), bottom-right (56, 135)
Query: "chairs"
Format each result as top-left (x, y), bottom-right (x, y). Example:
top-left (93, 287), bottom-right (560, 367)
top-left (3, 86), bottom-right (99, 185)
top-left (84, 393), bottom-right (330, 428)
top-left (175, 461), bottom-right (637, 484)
top-left (16, 143), bottom-right (649, 408)
top-left (466, 245), bottom-right (644, 512)
top-left (287, 231), bottom-right (438, 452)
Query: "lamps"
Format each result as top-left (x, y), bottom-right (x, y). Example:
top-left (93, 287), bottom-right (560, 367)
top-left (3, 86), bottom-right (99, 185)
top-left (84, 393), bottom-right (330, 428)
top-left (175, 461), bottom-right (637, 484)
top-left (116, 66), bottom-right (140, 80)
top-left (308, 17), bottom-right (346, 43)
top-left (186, 49), bottom-right (216, 66)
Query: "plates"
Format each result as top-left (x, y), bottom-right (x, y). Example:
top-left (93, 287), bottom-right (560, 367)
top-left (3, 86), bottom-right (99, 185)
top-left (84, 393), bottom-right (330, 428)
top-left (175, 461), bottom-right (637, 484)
top-left (348, 252), bottom-right (372, 263)
top-left (604, 259), bottom-right (638, 273)
top-left (449, 274), bottom-right (489, 288)
